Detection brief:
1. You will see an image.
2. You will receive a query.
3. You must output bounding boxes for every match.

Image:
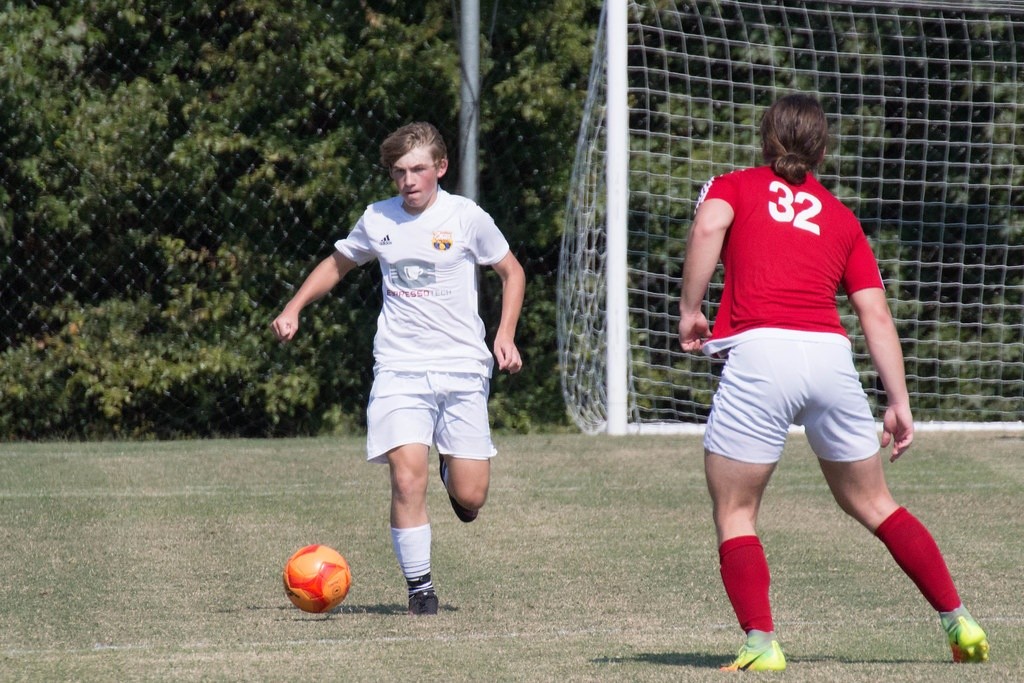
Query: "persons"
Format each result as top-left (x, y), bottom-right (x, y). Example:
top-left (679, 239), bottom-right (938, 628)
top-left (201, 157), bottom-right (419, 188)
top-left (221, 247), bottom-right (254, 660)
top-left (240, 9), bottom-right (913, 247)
top-left (677, 94), bottom-right (990, 669)
top-left (273, 123), bottom-right (528, 614)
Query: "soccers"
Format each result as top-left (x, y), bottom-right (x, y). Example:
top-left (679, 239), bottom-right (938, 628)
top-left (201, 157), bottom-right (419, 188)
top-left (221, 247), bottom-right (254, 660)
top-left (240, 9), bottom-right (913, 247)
top-left (283, 544), bottom-right (352, 614)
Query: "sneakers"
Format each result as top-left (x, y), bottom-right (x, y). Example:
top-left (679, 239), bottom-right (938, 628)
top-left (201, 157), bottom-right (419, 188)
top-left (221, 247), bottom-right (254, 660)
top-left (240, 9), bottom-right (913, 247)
top-left (439, 459), bottom-right (477, 522)
top-left (719, 641), bottom-right (787, 674)
top-left (940, 617), bottom-right (991, 664)
top-left (404, 575), bottom-right (439, 613)
